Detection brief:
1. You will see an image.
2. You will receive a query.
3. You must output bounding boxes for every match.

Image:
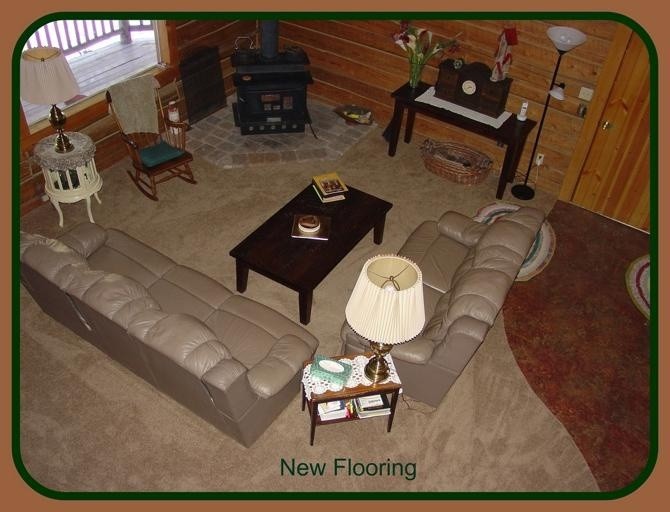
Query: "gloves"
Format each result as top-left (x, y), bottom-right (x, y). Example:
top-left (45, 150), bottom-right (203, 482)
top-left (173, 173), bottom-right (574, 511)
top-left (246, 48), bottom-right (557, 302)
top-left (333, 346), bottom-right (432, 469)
top-left (420, 136), bottom-right (494, 186)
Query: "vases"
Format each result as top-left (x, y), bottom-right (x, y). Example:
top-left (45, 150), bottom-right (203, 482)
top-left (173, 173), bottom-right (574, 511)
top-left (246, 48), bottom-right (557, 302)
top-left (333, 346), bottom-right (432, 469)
top-left (408, 57), bottom-right (425, 89)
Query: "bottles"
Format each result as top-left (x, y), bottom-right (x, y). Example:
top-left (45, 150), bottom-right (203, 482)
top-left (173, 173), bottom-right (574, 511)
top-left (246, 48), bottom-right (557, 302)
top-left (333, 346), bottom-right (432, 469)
top-left (168, 100), bottom-right (182, 135)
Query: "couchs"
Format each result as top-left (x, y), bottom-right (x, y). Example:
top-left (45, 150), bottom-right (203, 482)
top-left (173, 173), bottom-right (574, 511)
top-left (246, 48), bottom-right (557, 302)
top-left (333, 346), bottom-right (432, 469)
top-left (340, 206), bottom-right (545, 408)
top-left (19, 222), bottom-right (320, 449)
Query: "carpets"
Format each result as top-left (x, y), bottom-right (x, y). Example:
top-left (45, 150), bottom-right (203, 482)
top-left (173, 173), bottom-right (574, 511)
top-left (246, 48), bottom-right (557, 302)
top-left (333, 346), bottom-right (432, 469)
top-left (625, 254), bottom-right (651, 323)
top-left (471, 201), bottom-right (558, 282)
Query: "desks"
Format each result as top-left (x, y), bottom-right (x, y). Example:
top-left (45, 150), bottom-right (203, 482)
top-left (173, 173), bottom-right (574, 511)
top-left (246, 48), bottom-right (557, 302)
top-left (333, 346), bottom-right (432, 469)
top-left (300, 351), bottom-right (401, 446)
top-left (35, 132), bottom-right (104, 226)
top-left (388, 81), bottom-right (537, 199)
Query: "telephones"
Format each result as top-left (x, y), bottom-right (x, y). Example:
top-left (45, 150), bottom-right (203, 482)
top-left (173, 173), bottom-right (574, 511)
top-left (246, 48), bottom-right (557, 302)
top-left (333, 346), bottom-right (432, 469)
top-left (517, 101), bottom-right (529, 121)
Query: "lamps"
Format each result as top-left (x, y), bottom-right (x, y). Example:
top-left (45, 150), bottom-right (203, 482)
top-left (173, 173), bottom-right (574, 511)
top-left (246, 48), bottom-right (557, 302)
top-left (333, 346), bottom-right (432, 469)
top-left (510, 26), bottom-right (588, 200)
top-left (344, 253), bottom-right (428, 383)
top-left (20, 46), bottom-right (81, 152)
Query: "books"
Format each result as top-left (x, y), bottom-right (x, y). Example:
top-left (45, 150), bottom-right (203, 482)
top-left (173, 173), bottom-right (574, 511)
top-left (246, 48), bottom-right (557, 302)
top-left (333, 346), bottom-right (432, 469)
top-left (312, 172), bottom-right (350, 204)
top-left (317, 392), bottom-right (392, 420)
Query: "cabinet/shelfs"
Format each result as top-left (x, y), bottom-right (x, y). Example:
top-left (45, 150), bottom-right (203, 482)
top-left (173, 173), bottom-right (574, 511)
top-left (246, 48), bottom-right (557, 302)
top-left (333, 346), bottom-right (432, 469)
top-left (231, 72), bottom-right (314, 135)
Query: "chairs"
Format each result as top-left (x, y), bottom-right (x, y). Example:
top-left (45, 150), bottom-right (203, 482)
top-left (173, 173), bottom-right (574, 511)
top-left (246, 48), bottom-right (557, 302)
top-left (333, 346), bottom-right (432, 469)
top-left (105, 74), bottom-right (198, 200)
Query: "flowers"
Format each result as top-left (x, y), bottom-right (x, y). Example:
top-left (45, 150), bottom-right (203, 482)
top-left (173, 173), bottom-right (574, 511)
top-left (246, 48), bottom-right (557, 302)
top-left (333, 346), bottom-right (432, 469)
top-left (388, 19), bottom-right (463, 88)
